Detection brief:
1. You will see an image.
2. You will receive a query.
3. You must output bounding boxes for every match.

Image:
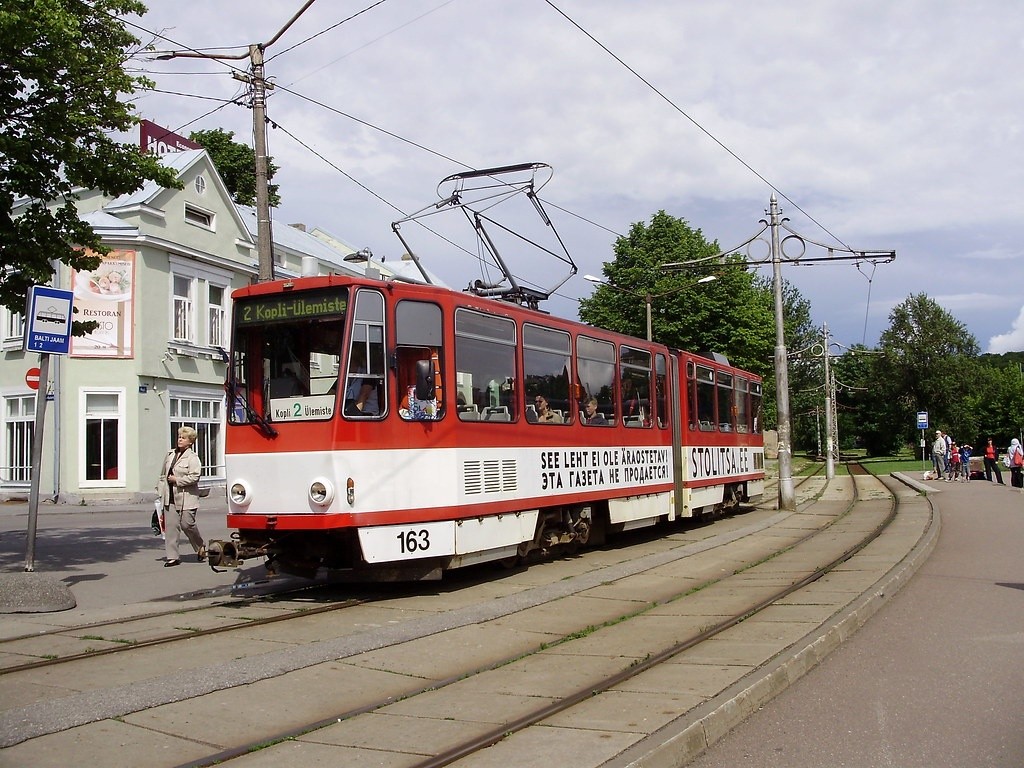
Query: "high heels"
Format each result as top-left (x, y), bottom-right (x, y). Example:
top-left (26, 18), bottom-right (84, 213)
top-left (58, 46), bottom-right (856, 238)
top-left (164, 558), bottom-right (181, 567)
top-left (198, 545), bottom-right (205, 561)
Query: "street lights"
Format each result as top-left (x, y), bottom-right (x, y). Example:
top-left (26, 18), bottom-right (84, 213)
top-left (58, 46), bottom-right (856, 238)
top-left (584, 275), bottom-right (718, 345)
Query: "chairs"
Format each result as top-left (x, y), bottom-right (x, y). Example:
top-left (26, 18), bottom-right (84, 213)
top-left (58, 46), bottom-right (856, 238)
top-left (454, 404), bottom-right (757, 434)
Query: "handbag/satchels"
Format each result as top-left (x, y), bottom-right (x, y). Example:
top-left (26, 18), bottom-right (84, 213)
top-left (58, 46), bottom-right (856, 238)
top-left (154, 497), bottom-right (165, 542)
top-left (1014, 452), bottom-right (1023, 466)
top-left (151, 509), bottom-right (162, 536)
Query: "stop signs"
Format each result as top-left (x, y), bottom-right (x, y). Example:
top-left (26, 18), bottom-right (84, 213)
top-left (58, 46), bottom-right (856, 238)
top-left (25, 368), bottom-right (40, 390)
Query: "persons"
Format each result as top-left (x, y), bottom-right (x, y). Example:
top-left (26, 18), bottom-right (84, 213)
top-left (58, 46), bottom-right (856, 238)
top-left (154, 425), bottom-right (206, 567)
top-left (938, 429), bottom-right (952, 473)
top-left (457, 391), bottom-right (468, 412)
top-left (507, 390), bottom-right (537, 423)
top-left (944, 442), bottom-right (960, 482)
top-left (582, 397), bottom-right (609, 426)
top-left (534, 393), bottom-right (563, 424)
top-left (328, 350), bottom-right (379, 417)
top-left (983, 438), bottom-right (1006, 486)
top-left (1006, 438), bottom-right (1024, 488)
top-left (931, 430), bottom-right (949, 481)
top-left (958, 443), bottom-right (973, 483)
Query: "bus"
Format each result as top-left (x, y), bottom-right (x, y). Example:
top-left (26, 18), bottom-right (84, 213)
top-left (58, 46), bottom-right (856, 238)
top-left (220, 273), bottom-right (766, 587)
